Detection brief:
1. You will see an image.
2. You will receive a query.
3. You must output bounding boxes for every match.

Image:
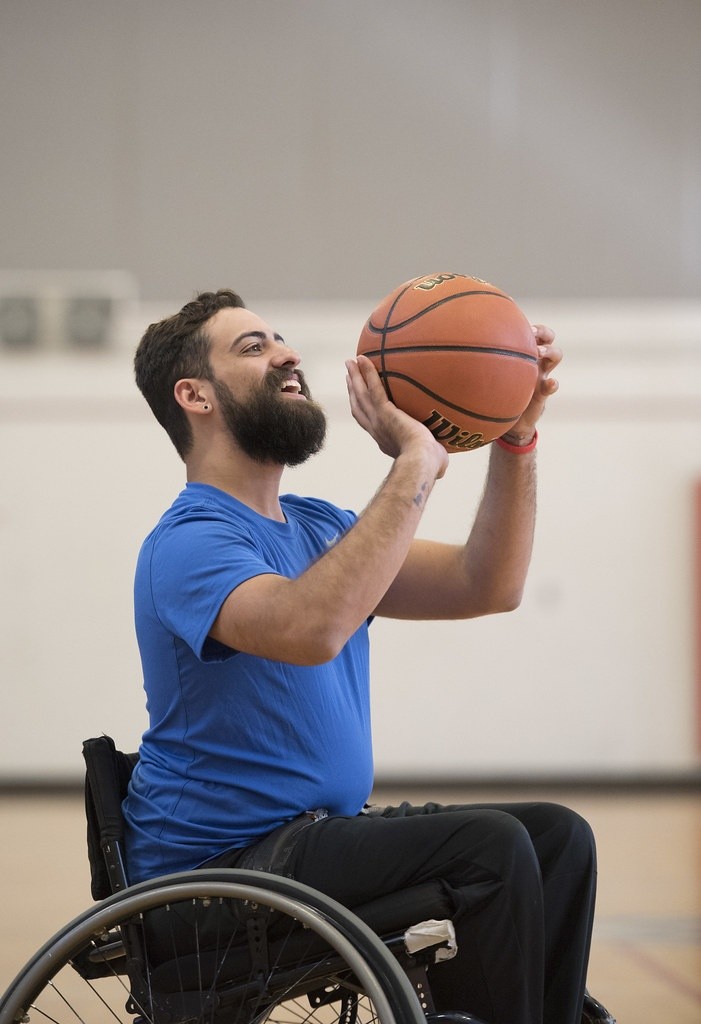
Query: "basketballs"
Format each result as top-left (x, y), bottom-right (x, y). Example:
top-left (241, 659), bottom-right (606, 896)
top-left (358, 271), bottom-right (543, 458)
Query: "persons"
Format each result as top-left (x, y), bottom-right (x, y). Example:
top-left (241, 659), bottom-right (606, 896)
top-left (120, 289), bottom-right (597, 1024)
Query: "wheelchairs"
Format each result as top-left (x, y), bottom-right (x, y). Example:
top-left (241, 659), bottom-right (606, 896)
top-left (0, 734), bottom-right (615, 1024)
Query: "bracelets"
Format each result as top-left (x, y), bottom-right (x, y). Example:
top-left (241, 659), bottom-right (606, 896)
top-left (495, 430), bottom-right (538, 454)
